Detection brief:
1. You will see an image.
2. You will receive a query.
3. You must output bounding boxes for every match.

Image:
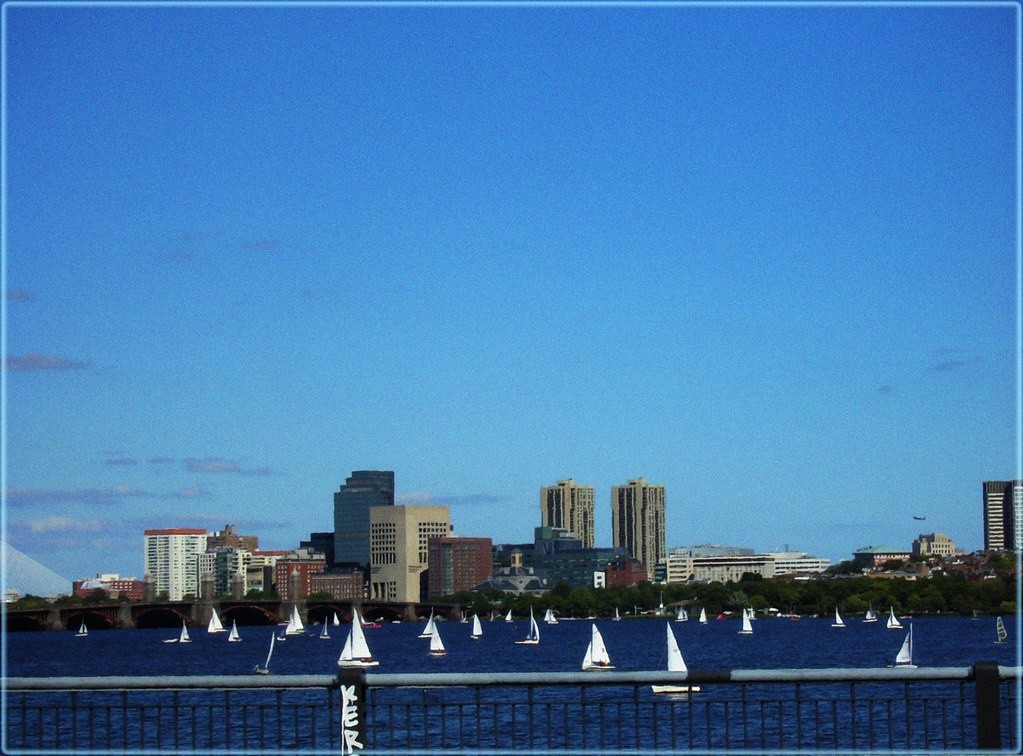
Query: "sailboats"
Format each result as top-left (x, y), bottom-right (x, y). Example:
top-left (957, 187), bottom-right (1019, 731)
top-left (336, 606), bottom-right (380, 671)
top-left (993, 616), bottom-right (1008, 644)
top-left (228, 619), bottom-right (243, 644)
top-left (320, 615), bottom-right (330, 639)
top-left (275, 605), bottom-right (306, 642)
top-left (699, 607), bottom-right (708, 626)
top-left (581, 623), bottom-right (617, 672)
top-left (417, 606), bottom-right (436, 638)
top-left (675, 606), bottom-right (689, 623)
top-left (831, 605), bottom-right (847, 628)
top-left (179, 620), bottom-right (193, 644)
top-left (737, 608), bottom-right (758, 635)
top-left (862, 609), bottom-right (878, 623)
top-left (886, 606), bottom-right (904, 628)
top-left (459, 605), bottom-right (561, 646)
top-left (427, 620), bottom-right (448, 656)
top-left (886, 622), bottom-right (919, 668)
top-left (208, 609), bottom-right (227, 635)
top-left (651, 621), bottom-right (701, 693)
top-left (74, 617), bottom-right (88, 637)
